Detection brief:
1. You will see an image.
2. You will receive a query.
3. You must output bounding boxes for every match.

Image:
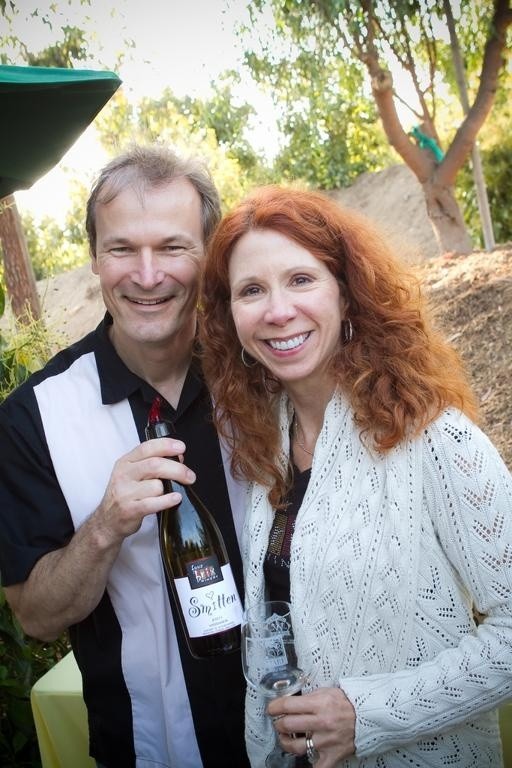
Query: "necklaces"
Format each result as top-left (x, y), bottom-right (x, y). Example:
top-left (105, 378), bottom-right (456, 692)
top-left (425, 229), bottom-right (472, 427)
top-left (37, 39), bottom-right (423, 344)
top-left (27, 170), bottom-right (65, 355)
top-left (292, 411), bottom-right (317, 456)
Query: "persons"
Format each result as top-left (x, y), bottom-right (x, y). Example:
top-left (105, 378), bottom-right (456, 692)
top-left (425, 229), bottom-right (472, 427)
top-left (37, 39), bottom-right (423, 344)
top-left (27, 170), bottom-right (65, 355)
top-left (191, 187), bottom-right (510, 768)
top-left (0, 147), bottom-right (251, 766)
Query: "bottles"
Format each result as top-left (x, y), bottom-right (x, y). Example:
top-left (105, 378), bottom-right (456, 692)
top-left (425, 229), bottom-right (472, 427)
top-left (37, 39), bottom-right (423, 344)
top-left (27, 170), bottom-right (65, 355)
top-left (143, 417), bottom-right (247, 664)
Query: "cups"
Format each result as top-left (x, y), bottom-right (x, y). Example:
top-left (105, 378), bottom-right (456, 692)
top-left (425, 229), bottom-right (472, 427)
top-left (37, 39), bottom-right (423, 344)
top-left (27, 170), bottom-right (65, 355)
top-left (240, 599), bottom-right (314, 768)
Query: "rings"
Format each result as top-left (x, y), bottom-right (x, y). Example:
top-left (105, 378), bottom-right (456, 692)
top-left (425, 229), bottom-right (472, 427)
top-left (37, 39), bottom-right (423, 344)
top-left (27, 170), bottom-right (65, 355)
top-left (305, 735), bottom-right (315, 751)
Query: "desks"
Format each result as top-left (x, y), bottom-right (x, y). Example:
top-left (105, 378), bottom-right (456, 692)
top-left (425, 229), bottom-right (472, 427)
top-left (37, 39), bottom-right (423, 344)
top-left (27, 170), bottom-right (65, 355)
top-left (28, 650), bottom-right (512, 767)
